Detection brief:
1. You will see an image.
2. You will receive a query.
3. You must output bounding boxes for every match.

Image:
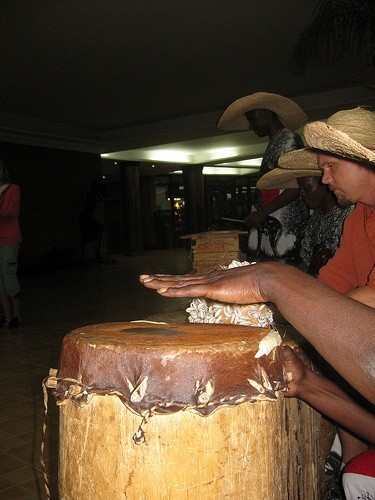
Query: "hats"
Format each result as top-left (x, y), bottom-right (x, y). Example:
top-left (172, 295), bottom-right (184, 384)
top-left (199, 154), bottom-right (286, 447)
top-left (278, 147), bottom-right (321, 170)
top-left (217, 92), bottom-right (308, 131)
top-left (304, 106), bottom-right (375, 166)
top-left (256, 167), bottom-right (324, 190)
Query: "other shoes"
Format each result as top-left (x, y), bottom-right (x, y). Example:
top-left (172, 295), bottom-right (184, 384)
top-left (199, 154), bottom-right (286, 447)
top-left (99, 257), bottom-right (118, 265)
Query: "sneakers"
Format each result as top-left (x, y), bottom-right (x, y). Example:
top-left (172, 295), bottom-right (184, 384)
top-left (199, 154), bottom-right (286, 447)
top-left (8, 317), bottom-right (24, 329)
top-left (0, 318), bottom-right (12, 328)
top-left (321, 452), bottom-right (346, 500)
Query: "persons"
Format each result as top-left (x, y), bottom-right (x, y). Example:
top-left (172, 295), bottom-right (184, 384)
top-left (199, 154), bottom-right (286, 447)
top-left (302, 106), bottom-right (375, 308)
top-left (254, 146), bottom-right (359, 278)
top-left (216, 88), bottom-right (314, 267)
top-left (136, 261), bottom-right (375, 500)
top-left (0, 165), bottom-right (25, 329)
